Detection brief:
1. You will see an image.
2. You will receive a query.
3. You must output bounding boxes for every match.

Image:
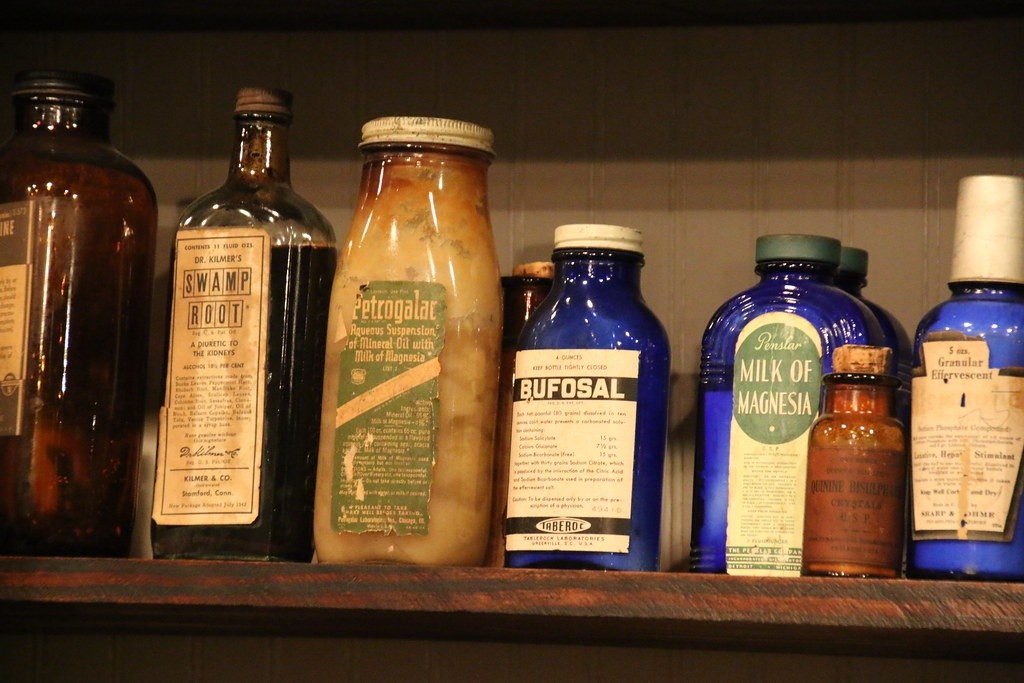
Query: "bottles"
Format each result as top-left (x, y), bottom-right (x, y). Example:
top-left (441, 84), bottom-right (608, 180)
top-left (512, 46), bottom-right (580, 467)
top-left (488, 274), bottom-right (556, 563)
top-left (803, 343), bottom-right (904, 580)
top-left (836, 248), bottom-right (918, 425)
top-left (149, 83), bottom-right (343, 564)
top-left (499, 223), bottom-right (671, 573)
top-left (689, 232), bottom-right (885, 577)
top-left (909, 175), bottom-right (1024, 582)
top-left (0, 65), bottom-right (158, 558)
top-left (311, 116), bottom-right (503, 565)
